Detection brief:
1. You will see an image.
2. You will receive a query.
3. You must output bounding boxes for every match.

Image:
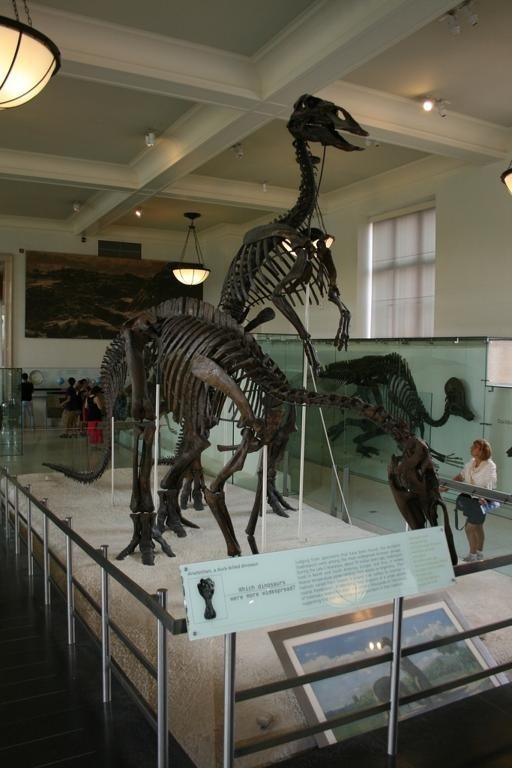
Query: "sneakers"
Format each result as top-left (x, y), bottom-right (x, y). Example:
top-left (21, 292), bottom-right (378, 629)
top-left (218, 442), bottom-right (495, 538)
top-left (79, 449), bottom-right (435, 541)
top-left (462, 552), bottom-right (484, 562)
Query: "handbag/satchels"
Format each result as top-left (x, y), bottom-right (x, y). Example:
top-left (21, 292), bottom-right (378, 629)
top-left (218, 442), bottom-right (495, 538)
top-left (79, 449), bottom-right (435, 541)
top-left (456, 493), bottom-right (472, 512)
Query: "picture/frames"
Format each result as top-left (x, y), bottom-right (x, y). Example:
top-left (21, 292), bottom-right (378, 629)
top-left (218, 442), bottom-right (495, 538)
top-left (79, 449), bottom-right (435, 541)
top-left (267, 589), bottom-right (510, 748)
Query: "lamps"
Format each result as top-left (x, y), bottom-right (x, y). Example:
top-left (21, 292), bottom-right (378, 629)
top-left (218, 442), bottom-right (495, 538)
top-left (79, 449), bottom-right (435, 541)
top-left (171, 212), bottom-right (211, 290)
top-left (279, 153), bottom-right (335, 260)
top-left (0, 0), bottom-right (61, 116)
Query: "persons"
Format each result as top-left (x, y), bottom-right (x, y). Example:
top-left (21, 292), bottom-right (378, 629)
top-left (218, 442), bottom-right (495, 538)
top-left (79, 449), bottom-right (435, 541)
top-left (17, 373), bottom-right (35, 431)
top-left (439, 439), bottom-right (497, 563)
top-left (57, 376), bottom-right (104, 451)
